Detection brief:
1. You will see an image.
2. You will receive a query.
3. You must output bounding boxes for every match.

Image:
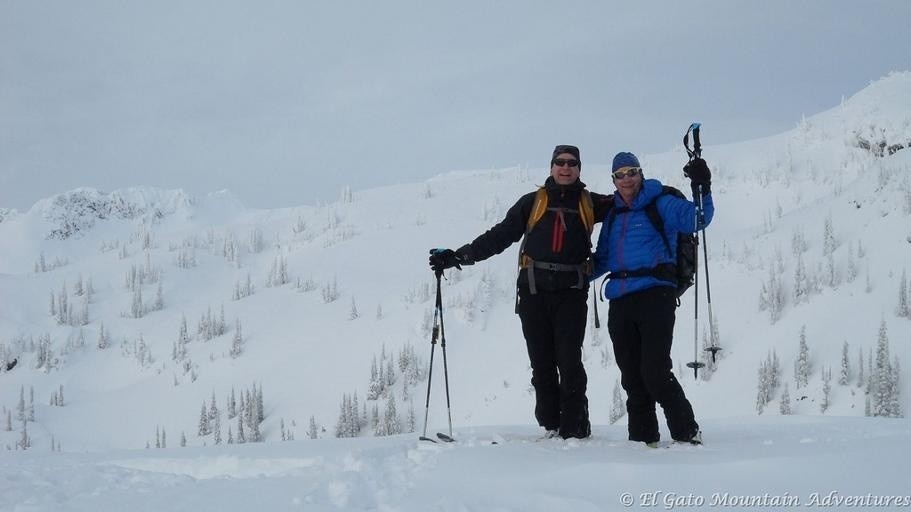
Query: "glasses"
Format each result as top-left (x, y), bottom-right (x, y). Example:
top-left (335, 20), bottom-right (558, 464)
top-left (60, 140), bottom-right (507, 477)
top-left (611, 166), bottom-right (641, 180)
top-left (553, 158), bottom-right (579, 168)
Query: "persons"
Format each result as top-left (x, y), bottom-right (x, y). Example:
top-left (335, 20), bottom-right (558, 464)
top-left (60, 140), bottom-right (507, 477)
top-left (429, 145), bottom-right (615, 439)
top-left (589, 152), bottom-right (714, 448)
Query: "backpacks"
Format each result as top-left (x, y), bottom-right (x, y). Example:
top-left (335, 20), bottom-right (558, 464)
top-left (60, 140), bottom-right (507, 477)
top-left (599, 185), bottom-right (700, 308)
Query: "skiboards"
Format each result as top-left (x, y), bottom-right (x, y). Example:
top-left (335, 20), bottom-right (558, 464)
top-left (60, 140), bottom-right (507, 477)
top-left (419, 432), bottom-right (457, 442)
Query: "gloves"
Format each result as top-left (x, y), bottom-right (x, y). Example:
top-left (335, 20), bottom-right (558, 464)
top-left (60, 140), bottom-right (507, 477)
top-left (428, 248), bottom-right (463, 271)
top-left (683, 158), bottom-right (712, 199)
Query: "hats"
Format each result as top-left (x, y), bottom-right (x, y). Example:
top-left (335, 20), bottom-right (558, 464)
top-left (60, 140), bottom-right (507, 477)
top-left (552, 144), bottom-right (580, 161)
top-left (612, 151), bottom-right (641, 173)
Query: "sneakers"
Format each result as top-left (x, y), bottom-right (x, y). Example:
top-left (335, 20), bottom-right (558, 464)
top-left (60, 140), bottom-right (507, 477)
top-left (664, 430), bottom-right (703, 448)
top-left (534, 430), bottom-right (558, 443)
